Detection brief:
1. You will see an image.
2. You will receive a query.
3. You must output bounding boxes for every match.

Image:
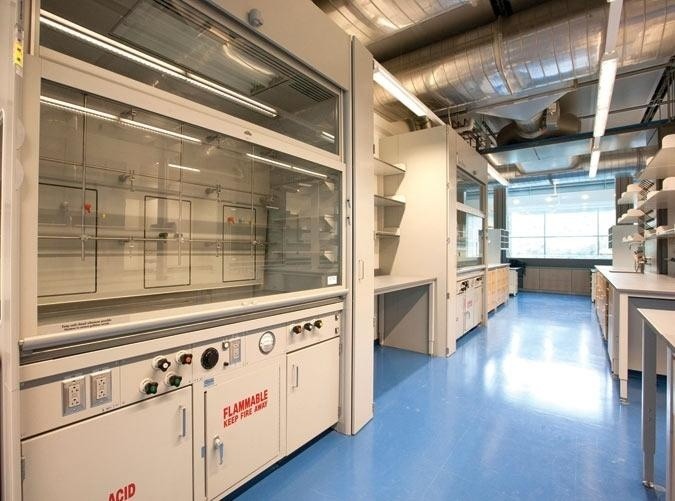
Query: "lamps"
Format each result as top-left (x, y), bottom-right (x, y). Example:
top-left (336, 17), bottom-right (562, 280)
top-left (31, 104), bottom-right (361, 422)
top-left (373, 68), bottom-right (427, 117)
top-left (594, 52), bottom-right (620, 139)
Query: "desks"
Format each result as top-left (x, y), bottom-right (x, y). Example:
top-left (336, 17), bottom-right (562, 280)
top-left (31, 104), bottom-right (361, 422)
top-left (632, 305), bottom-right (673, 501)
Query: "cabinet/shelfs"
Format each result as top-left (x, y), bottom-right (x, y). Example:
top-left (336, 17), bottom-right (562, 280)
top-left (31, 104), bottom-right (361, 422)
top-left (374, 152), bottom-right (406, 278)
top-left (591, 133), bottom-right (673, 406)
top-left (486, 227), bottom-right (527, 317)
